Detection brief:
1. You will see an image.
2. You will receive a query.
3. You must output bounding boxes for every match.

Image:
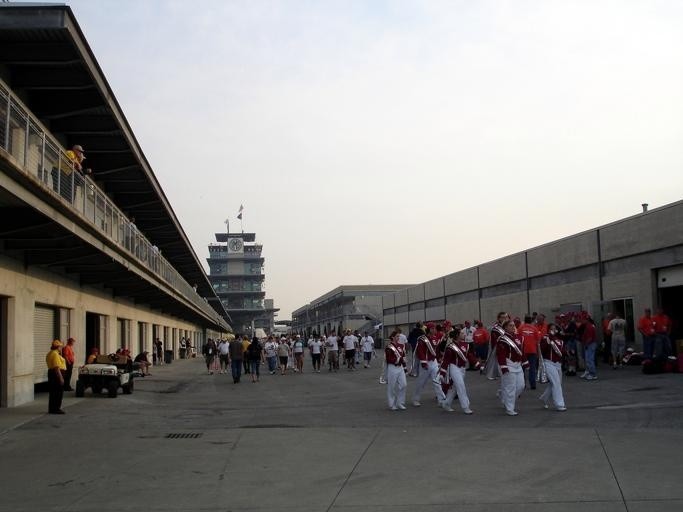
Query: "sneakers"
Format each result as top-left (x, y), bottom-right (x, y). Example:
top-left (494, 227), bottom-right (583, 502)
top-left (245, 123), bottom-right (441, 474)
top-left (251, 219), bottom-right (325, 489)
top-left (539, 398), bottom-right (567, 411)
top-left (433, 397), bottom-right (472, 414)
top-left (506, 410), bottom-right (517, 416)
top-left (390, 401), bottom-right (419, 409)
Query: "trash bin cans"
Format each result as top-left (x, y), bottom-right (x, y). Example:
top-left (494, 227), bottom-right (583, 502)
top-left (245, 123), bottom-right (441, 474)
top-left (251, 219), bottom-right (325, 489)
top-left (180, 348), bottom-right (187, 359)
top-left (165, 350), bottom-right (172, 364)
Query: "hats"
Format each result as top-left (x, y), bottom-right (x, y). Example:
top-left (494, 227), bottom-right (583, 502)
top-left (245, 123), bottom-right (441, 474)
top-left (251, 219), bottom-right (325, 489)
top-left (52, 340), bottom-right (63, 345)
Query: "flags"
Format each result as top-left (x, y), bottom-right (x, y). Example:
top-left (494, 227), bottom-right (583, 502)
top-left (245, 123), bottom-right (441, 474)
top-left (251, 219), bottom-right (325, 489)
top-left (235, 213), bottom-right (241, 220)
top-left (223, 219), bottom-right (229, 230)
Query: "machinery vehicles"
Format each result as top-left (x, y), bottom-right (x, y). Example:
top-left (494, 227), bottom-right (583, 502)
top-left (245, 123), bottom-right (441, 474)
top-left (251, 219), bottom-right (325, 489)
top-left (75, 353), bottom-right (136, 399)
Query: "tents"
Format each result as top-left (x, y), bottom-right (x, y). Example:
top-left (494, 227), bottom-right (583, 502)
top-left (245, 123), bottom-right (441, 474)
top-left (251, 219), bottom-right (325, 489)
top-left (237, 204), bottom-right (243, 212)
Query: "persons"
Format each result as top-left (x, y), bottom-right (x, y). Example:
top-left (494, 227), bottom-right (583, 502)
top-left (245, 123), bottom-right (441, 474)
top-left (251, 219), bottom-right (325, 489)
top-left (202, 328), bottom-right (376, 384)
top-left (86, 337), bottom-right (193, 376)
top-left (45, 340), bottom-right (66, 414)
top-left (378, 307), bottom-right (672, 416)
top-left (119, 213), bottom-right (208, 308)
top-left (61, 338), bottom-right (74, 391)
top-left (62, 153), bottom-right (92, 205)
top-left (50, 145), bottom-right (83, 197)
